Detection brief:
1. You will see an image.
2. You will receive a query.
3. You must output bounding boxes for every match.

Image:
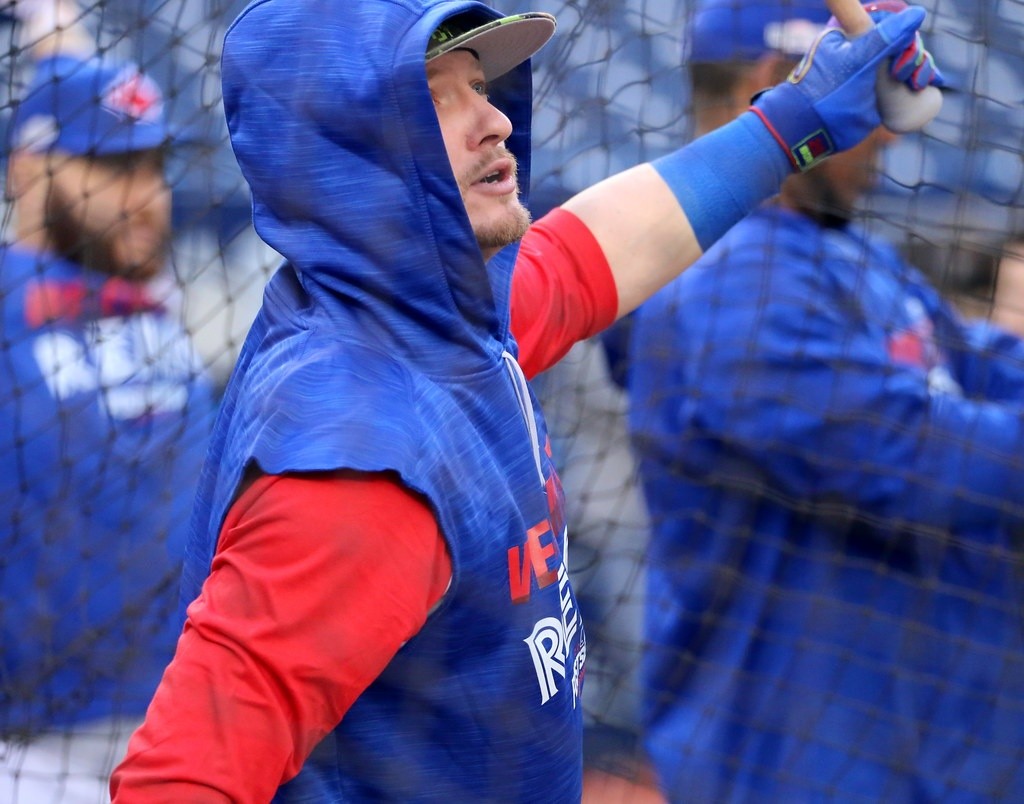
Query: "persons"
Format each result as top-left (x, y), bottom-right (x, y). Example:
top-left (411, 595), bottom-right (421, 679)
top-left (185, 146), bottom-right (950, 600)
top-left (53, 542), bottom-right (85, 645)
top-left (623, 2), bottom-right (1024, 803)
top-left (948, 235), bottom-right (1024, 346)
top-left (1, 55), bottom-right (222, 804)
top-left (110, 0), bottom-right (949, 803)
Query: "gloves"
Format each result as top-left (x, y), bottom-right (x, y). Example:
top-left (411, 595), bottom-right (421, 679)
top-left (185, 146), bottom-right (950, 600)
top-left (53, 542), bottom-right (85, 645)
top-left (746, 1), bottom-right (943, 172)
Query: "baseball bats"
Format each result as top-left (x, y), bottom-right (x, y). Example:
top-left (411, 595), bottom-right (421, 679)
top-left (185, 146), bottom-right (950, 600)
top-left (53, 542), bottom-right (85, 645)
top-left (824, 1), bottom-right (943, 135)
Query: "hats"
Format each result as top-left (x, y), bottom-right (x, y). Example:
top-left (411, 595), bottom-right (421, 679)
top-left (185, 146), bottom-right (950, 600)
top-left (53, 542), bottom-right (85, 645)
top-left (680, 0), bottom-right (837, 66)
top-left (425, 10), bottom-right (557, 79)
top-left (16, 57), bottom-right (221, 169)
